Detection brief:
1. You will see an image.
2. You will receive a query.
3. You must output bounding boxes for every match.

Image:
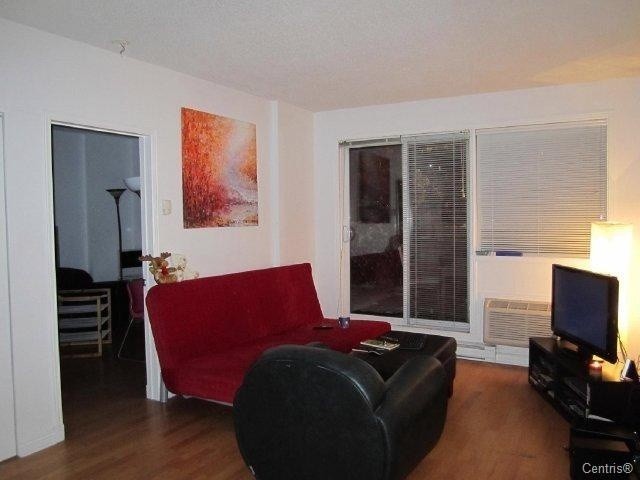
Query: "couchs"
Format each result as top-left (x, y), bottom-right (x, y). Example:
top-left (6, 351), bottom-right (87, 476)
top-left (351, 253), bottom-right (441, 318)
top-left (145, 263), bottom-right (391, 408)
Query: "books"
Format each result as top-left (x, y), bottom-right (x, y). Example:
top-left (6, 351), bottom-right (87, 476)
top-left (360, 339), bottom-right (401, 351)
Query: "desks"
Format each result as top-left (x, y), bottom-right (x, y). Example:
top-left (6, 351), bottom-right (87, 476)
top-left (528, 338), bottom-right (632, 427)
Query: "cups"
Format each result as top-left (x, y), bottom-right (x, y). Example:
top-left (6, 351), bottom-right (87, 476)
top-left (338, 314), bottom-right (351, 328)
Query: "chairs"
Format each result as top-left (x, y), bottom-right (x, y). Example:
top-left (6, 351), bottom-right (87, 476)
top-left (232, 342), bottom-right (448, 480)
top-left (117, 278), bottom-right (145, 360)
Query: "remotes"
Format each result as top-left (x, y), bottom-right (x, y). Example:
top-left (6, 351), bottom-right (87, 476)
top-left (351, 345), bottom-right (383, 355)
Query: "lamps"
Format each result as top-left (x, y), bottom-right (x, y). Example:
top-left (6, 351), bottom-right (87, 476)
top-left (123, 176), bottom-right (140, 198)
top-left (591, 222), bottom-right (640, 361)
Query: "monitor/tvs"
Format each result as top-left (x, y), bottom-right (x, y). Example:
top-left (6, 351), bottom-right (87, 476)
top-left (551, 264), bottom-right (619, 364)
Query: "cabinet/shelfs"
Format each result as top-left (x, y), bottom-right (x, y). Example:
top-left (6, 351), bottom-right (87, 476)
top-left (57, 288), bottom-right (112, 359)
